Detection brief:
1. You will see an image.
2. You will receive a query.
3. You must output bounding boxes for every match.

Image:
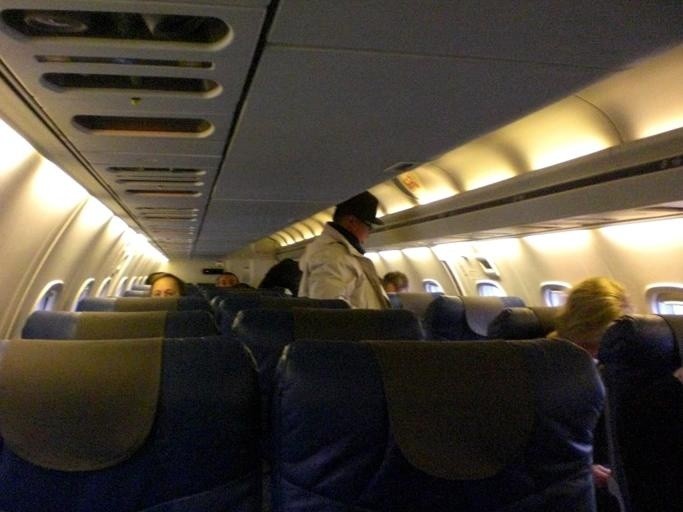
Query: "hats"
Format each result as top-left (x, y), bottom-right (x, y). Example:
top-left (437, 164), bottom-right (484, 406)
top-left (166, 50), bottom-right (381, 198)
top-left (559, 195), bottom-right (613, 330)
top-left (335, 190), bottom-right (385, 227)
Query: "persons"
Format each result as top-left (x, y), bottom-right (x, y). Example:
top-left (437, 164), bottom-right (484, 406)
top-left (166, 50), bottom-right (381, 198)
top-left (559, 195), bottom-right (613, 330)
top-left (258, 258), bottom-right (300, 291)
top-left (215, 272), bottom-right (239, 288)
top-left (545, 275), bottom-right (631, 511)
top-left (297, 191), bottom-right (392, 309)
top-left (383, 271), bottom-right (408, 292)
top-left (150, 274), bottom-right (185, 297)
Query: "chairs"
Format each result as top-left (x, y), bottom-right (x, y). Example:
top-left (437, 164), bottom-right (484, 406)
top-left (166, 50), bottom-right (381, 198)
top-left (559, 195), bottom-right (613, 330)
top-left (1, 273), bottom-right (681, 511)
